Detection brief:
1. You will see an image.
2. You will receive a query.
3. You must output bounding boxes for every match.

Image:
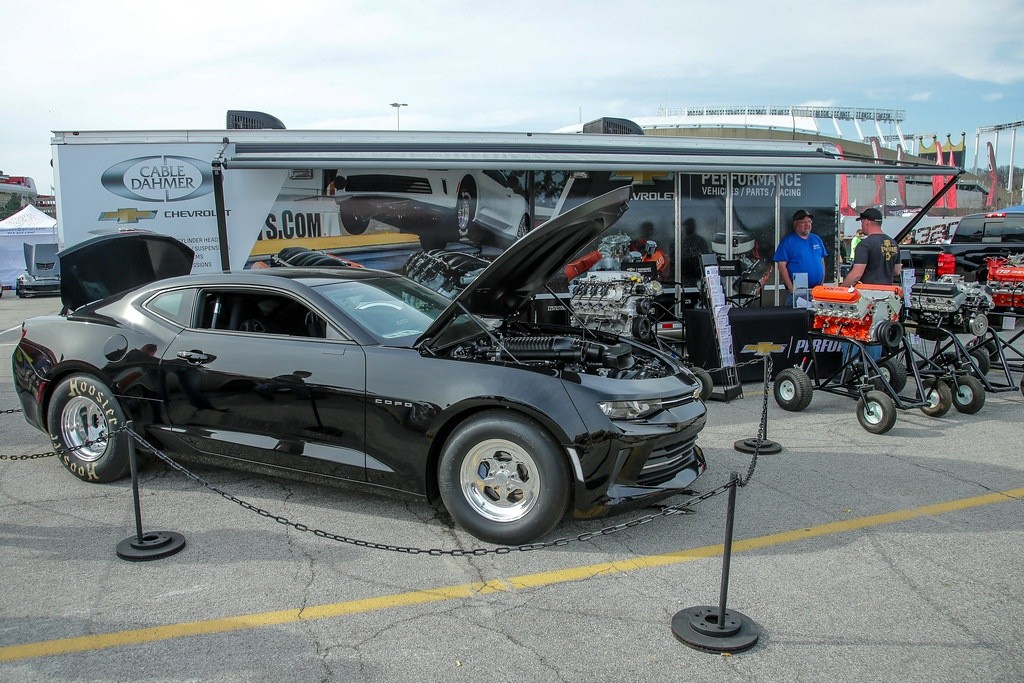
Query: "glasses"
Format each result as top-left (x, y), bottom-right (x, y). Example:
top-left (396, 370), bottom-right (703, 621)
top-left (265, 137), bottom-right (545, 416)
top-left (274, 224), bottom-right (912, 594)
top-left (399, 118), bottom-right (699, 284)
top-left (858, 233), bottom-right (862, 234)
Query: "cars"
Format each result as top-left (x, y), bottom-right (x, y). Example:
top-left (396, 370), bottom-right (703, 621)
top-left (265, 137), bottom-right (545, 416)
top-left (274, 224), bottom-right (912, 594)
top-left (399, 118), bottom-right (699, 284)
top-left (333, 169), bottom-right (530, 252)
top-left (15, 243), bottom-right (61, 298)
top-left (12, 185), bottom-right (708, 545)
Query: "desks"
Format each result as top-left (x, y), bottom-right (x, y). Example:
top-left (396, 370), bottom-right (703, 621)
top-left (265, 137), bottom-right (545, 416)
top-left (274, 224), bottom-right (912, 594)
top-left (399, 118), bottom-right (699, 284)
top-left (684, 306), bottom-right (841, 386)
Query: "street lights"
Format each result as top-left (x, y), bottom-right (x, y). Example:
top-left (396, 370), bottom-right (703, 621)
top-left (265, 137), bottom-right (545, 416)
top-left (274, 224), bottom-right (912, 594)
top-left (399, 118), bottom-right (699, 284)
top-left (389, 103), bottom-right (408, 131)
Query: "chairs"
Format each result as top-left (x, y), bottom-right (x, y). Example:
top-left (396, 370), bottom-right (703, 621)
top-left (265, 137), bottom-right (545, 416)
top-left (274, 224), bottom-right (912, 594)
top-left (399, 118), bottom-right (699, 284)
top-left (228, 303), bottom-right (271, 332)
top-left (290, 299), bottom-right (327, 338)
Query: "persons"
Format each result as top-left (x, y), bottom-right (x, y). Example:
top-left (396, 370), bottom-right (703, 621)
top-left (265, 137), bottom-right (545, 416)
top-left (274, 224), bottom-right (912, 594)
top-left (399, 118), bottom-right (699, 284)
top-left (902, 232), bottom-right (916, 269)
top-left (839, 208), bottom-right (902, 361)
top-left (840, 231), bottom-right (847, 264)
top-left (773, 210), bottom-right (828, 306)
top-left (936, 230), bottom-right (952, 244)
top-left (850, 229), bottom-right (863, 266)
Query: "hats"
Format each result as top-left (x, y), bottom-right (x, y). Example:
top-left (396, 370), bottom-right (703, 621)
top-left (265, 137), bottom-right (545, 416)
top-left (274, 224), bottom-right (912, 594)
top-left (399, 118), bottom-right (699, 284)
top-left (793, 210), bottom-right (814, 222)
top-left (856, 208), bottom-right (882, 222)
top-left (682, 218), bottom-right (694, 225)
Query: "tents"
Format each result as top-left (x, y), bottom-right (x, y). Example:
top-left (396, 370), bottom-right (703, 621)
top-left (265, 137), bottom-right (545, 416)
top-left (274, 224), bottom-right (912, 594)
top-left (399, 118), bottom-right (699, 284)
top-left (0, 204), bottom-right (58, 291)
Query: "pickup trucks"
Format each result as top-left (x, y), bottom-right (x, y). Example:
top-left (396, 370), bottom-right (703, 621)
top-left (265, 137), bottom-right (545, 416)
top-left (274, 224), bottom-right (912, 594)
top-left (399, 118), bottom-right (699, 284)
top-left (840, 212), bottom-right (1024, 308)
